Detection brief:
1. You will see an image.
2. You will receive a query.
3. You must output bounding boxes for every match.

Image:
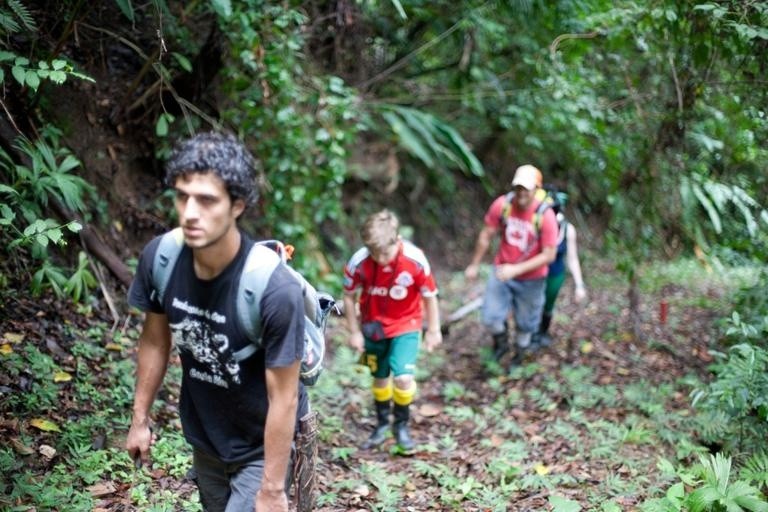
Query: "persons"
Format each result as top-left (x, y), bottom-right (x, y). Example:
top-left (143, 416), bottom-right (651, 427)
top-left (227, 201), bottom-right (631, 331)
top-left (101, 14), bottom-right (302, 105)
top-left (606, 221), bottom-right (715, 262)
top-left (124, 126), bottom-right (305, 512)
top-left (463, 164), bottom-right (556, 371)
top-left (529, 190), bottom-right (588, 347)
top-left (343, 210), bottom-right (443, 457)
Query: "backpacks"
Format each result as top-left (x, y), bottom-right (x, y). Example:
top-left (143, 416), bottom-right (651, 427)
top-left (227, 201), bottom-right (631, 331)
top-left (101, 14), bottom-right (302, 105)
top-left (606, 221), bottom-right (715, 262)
top-left (499, 187), bottom-right (560, 232)
top-left (153, 227), bottom-right (334, 386)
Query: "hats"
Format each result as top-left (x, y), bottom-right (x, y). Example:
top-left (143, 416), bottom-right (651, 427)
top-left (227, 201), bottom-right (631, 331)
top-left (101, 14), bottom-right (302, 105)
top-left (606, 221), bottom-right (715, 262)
top-left (512, 165), bottom-right (543, 190)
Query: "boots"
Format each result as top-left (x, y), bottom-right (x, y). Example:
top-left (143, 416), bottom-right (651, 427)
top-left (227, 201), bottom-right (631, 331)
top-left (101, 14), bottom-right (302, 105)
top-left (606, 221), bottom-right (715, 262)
top-left (366, 383), bottom-right (392, 445)
top-left (393, 381), bottom-right (417, 456)
top-left (493, 313), bottom-right (551, 364)
